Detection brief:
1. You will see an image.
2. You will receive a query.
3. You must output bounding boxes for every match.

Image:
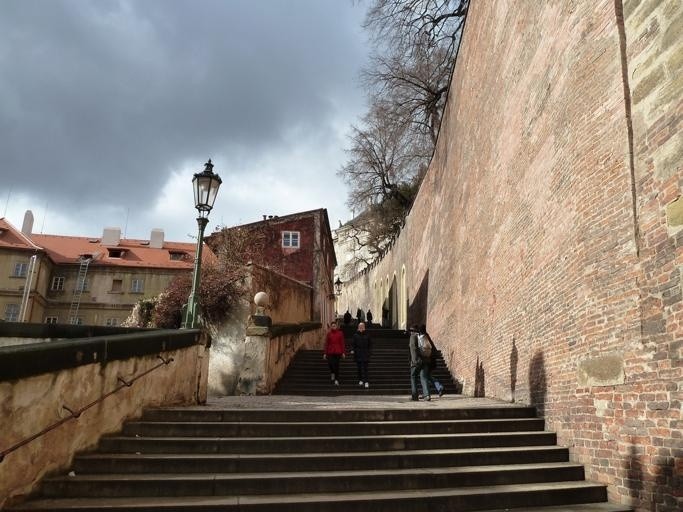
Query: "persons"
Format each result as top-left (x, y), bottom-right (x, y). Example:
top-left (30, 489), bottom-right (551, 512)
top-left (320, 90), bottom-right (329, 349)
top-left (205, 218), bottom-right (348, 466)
top-left (321, 321), bottom-right (347, 387)
top-left (366, 309), bottom-right (372, 326)
top-left (415, 325), bottom-right (446, 399)
top-left (356, 308), bottom-right (362, 325)
top-left (408, 323), bottom-right (432, 401)
top-left (349, 322), bottom-right (374, 388)
top-left (344, 310), bottom-right (352, 328)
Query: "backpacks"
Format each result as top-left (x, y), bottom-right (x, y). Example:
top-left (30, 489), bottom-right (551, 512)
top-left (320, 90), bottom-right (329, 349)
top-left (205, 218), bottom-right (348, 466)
top-left (416, 332), bottom-right (433, 358)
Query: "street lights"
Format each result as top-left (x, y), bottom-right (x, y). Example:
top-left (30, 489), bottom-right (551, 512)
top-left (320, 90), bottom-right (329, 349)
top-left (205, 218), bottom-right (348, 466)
top-left (180, 158), bottom-right (223, 331)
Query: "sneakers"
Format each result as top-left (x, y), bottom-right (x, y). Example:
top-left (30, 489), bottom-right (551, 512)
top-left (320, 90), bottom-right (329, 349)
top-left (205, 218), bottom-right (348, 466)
top-left (410, 392), bottom-right (431, 401)
top-left (330, 373), bottom-right (341, 388)
top-left (358, 380), bottom-right (369, 389)
top-left (438, 385), bottom-right (445, 397)
